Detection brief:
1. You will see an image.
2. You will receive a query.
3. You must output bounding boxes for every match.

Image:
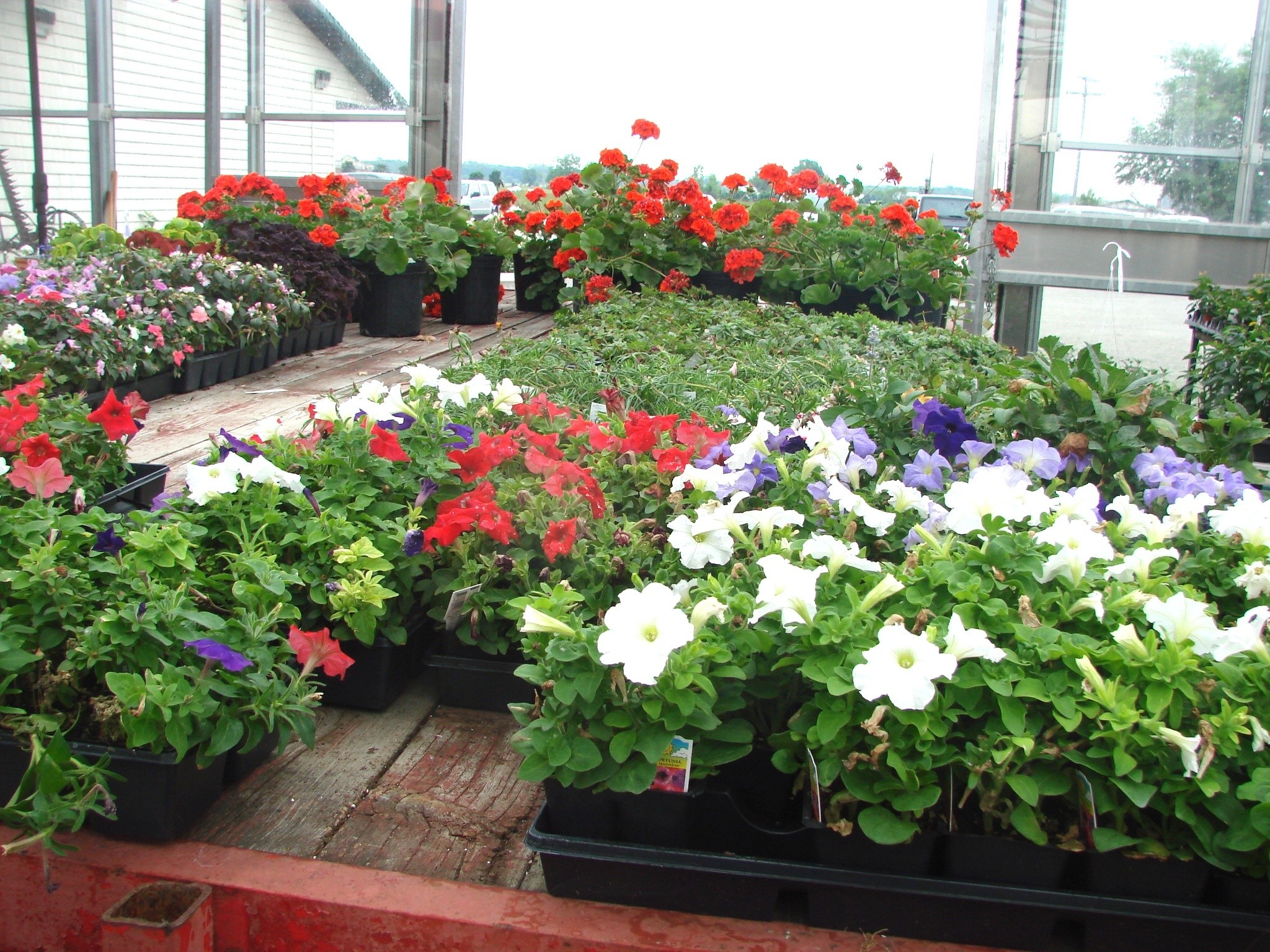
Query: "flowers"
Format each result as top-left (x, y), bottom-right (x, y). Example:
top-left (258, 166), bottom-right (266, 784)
top-left (0, 116), bottom-right (1270, 884)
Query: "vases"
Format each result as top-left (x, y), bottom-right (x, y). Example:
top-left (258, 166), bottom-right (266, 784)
top-left (692, 270), bottom-right (762, 306)
top-left (82, 464), bottom-right (170, 518)
top-left (357, 261), bottom-right (426, 337)
top-left (514, 253), bottom-right (565, 312)
top-left (794, 277), bottom-right (942, 327)
top-left (0, 695), bottom-right (280, 845)
top-left (440, 255), bottom-right (505, 324)
top-left (51, 368), bottom-right (174, 413)
top-left (271, 608), bottom-right (430, 713)
top-left (183, 334), bottom-right (283, 391)
top-left (426, 632), bottom-right (544, 715)
top-left (280, 318), bottom-right (346, 357)
top-left (521, 773), bottom-right (1270, 952)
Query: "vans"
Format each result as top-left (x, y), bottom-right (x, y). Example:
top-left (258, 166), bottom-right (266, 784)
top-left (460, 180), bottom-right (500, 218)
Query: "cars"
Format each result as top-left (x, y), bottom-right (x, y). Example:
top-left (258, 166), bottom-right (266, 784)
top-left (337, 172), bottom-right (404, 180)
top-left (911, 193), bottom-right (975, 240)
